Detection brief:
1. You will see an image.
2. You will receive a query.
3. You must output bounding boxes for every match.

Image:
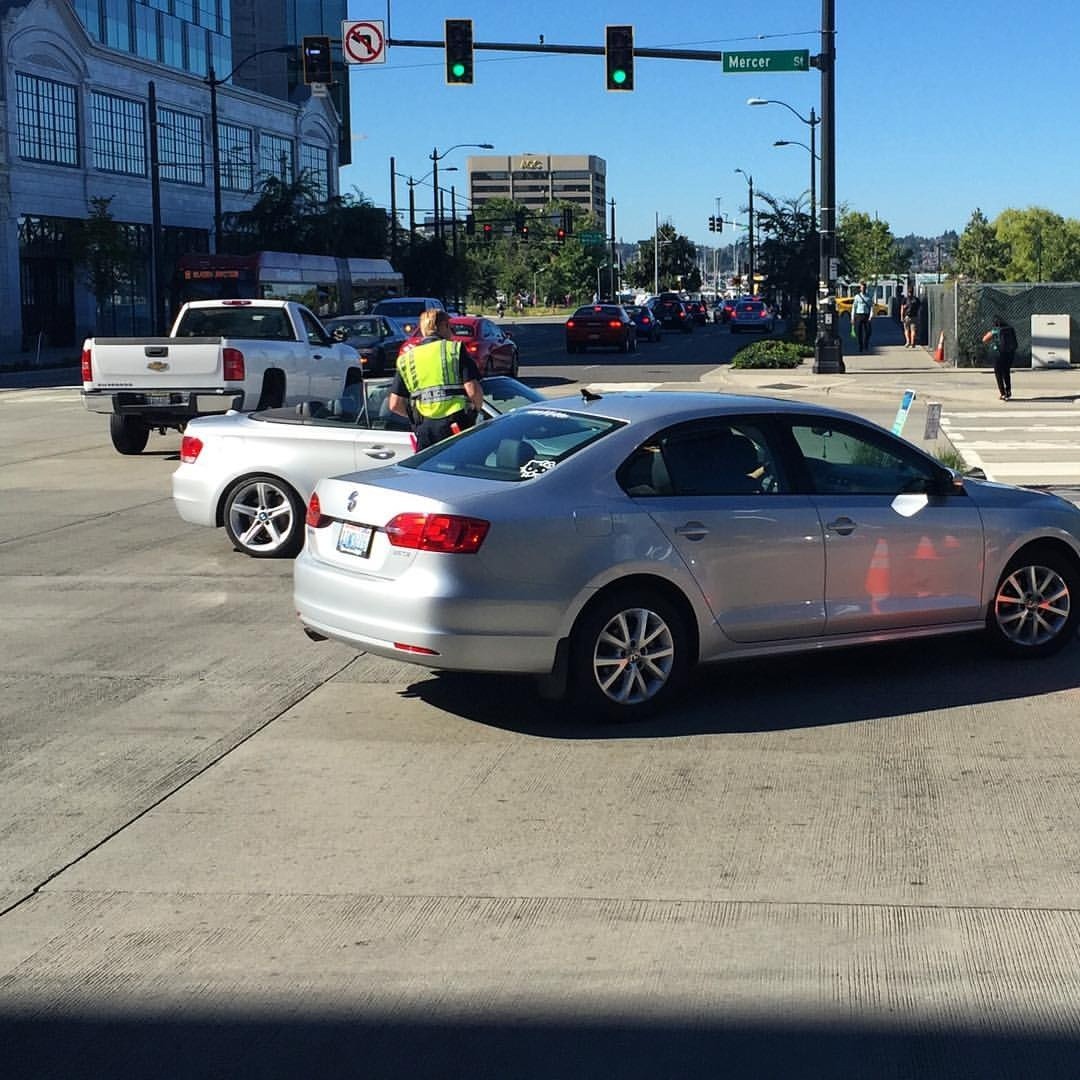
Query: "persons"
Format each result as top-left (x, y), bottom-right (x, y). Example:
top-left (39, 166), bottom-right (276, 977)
top-left (389, 309), bottom-right (483, 455)
top-left (850, 282), bottom-right (874, 355)
top-left (564, 291), bottom-right (573, 309)
top-left (495, 290), bottom-right (536, 318)
top-left (981, 315), bottom-right (1013, 401)
top-left (900, 289), bottom-right (922, 349)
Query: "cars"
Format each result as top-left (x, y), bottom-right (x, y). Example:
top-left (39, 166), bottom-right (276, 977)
top-left (713, 294), bottom-right (779, 334)
top-left (564, 292), bottom-right (710, 353)
top-left (831, 295), bottom-right (890, 320)
top-left (293, 387), bottom-right (1080, 724)
top-left (312, 295), bottom-right (520, 379)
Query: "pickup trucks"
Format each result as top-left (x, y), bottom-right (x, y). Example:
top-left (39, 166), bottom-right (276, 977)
top-left (78, 298), bottom-right (370, 455)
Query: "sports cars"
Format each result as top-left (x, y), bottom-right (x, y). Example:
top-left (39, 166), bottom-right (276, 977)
top-left (173, 375), bottom-right (554, 558)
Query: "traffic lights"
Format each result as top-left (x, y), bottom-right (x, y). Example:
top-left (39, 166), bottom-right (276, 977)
top-left (300, 33), bottom-right (333, 85)
top-left (443, 19), bottom-right (475, 84)
top-left (482, 224), bottom-right (493, 241)
top-left (603, 23), bottom-right (637, 92)
top-left (521, 227), bottom-right (528, 240)
top-left (708, 216), bottom-right (723, 232)
top-left (557, 229), bottom-right (565, 245)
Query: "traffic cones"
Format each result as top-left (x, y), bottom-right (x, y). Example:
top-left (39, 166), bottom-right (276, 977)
top-left (936, 330), bottom-right (947, 361)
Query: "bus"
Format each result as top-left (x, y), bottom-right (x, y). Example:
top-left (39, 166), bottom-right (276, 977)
top-left (170, 251), bottom-right (410, 328)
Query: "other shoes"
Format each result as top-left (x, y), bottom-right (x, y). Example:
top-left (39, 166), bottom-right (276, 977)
top-left (905, 343), bottom-right (910, 348)
top-left (1005, 392), bottom-right (1011, 398)
top-left (1000, 395), bottom-right (1007, 400)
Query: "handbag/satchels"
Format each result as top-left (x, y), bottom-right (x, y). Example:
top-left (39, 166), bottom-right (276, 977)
top-left (850, 323), bottom-right (856, 337)
top-left (866, 321), bottom-right (872, 337)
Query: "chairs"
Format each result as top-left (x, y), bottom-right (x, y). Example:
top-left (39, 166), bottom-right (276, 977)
top-left (496, 438), bottom-right (538, 469)
top-left (658, 422), bottom-right (766, 496)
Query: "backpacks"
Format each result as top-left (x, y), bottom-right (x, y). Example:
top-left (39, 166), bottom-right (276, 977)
top-left (999, 326), bottom-right (1018, 354)
top-left (905, 295), bottom-right (920, 318)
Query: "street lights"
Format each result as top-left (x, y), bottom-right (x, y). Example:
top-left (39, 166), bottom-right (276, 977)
top-left (429, 143), bottom-right (495, 239)
top-left (746, 94), bottom-right (821, 340)
top-left (205, 45), bottom-right (298, 254)
top-left (406, 166), bottom-right (459, 252)
top-left (734, 167), bottom-right (753, 294)
